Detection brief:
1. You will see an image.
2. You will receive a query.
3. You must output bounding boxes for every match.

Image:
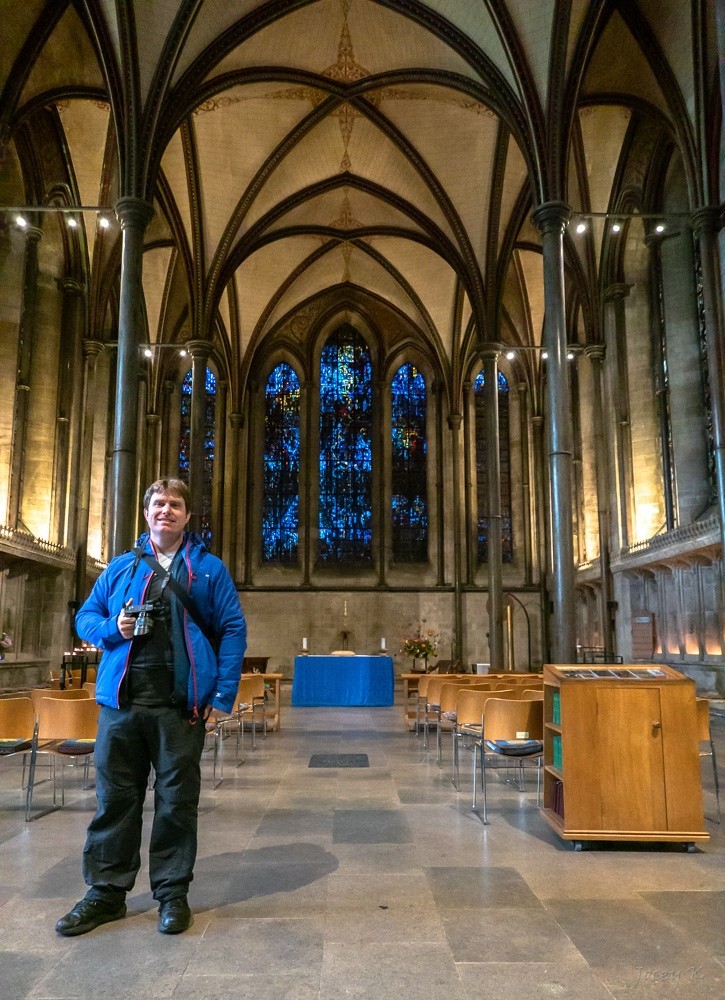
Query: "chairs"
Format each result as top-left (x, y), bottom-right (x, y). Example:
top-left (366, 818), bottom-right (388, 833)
top-left (413, 674), bottom-right (544, 825)
top-left (696, 697), bottom-right (723, 825)
top-left (0, 667), bottom-right (273, 823)
top-left (332, 650), bottom-right (355, 656)
top-left (426, 659), bottom-right (463, 674)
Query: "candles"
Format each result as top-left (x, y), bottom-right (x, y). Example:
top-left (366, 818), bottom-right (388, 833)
top-left (380, 637), bottom-right (385, 650)
top-left (302, 638), bottom-right (308, 650)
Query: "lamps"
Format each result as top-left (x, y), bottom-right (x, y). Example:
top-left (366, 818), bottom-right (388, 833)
top-left (576, 213), bottom-right (587, 233)
top-left (96, 212), bottom-right (109, 227)
top-left (63, 212), bottom-right (77, 227)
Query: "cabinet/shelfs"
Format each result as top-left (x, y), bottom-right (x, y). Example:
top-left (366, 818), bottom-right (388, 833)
top-left (540, 662), bottom-right (712, 853)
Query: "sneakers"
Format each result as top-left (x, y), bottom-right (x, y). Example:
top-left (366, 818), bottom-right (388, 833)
top-left (56, 898), bottom-right (127, 935)
top-left (158, 898), bottom-right (190, 933)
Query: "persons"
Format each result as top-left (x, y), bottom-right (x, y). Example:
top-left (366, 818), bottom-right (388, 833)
top-left (0, 632), bottom-right (12, 660)
top-left (55, 478), bottom-right (248, 936)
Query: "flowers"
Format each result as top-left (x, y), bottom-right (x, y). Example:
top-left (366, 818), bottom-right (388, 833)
top-left (0, 632), bottom-right (14, 649)
top-left (396, 617), bottom-right (440, 659)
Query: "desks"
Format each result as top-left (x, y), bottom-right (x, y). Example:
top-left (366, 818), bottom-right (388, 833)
top-left (291, 655), bottom-right (395, 708)
top-left (241, 673), bottom-right (291, 732)
top-left (400, 673), bottom-right (544, 732)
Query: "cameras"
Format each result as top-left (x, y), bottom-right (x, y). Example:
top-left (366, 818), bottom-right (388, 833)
top-left (125, 605), bottom-right (157, 636)
